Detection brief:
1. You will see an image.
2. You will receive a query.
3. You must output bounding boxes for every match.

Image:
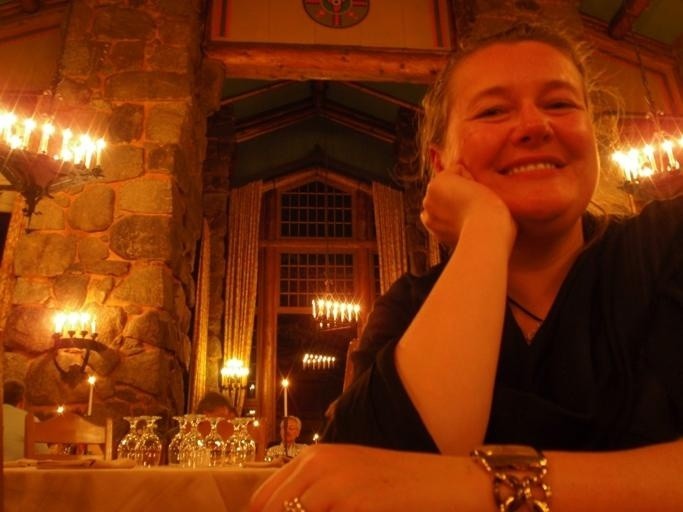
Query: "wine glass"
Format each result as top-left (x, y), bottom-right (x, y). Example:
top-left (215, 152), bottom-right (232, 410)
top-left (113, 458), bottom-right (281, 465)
top-left (115, 414), bottom-right (258, 469)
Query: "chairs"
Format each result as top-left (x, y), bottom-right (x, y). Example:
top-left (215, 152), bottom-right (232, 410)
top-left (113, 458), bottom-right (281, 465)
top-left (23, 410), bottom-right (268, 463)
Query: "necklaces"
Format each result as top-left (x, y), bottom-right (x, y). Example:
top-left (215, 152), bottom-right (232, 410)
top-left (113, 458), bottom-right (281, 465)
top-left (506, 294), bottom-right (546, 325)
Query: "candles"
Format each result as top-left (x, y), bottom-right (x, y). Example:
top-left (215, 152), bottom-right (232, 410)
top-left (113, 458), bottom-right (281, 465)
top-left (49, 308), bottom-right (289, 419)
top-left (605, 135), bottom-right (683, 183)
top-left (308, 294), bottom-right (364, 330)
top-left (0, 107), bottom-right (103, 174)
top-left (299, 352), bottom-right (338, 371)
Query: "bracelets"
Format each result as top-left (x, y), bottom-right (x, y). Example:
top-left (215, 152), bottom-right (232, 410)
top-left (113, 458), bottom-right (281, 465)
top-left (471, 444), bottom-right (551, 512)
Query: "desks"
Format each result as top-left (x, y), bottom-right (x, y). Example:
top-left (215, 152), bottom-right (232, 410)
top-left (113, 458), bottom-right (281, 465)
top-left (0, 459), bottom-right (282, 512)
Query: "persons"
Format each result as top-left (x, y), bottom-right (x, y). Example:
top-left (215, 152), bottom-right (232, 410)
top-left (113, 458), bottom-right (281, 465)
top-left (160, 392), bottom-right (230, 466)
top-left (254, 412), bottom-right (312, 463)
top-left (247, 11), bottom-right (682, 511)
top-left (220, 405), bottom-right (240, 436)
top-left (3, 378), bottom-right (49, 464)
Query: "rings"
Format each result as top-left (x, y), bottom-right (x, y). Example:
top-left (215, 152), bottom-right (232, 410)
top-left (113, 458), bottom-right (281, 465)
top-left (282, 497), bottom-right (303, 511)
